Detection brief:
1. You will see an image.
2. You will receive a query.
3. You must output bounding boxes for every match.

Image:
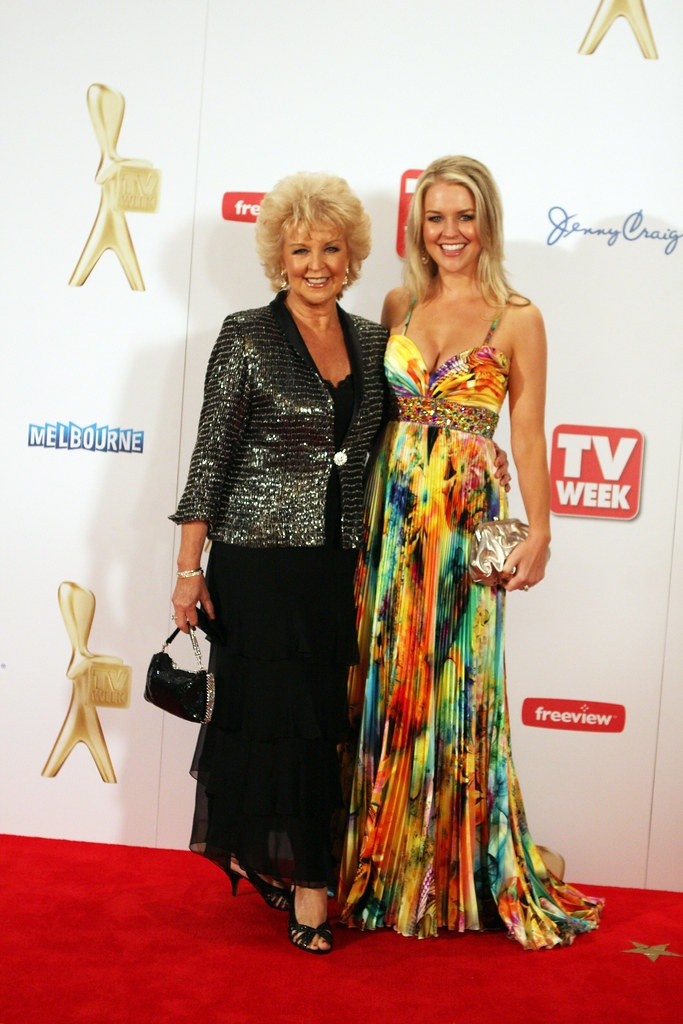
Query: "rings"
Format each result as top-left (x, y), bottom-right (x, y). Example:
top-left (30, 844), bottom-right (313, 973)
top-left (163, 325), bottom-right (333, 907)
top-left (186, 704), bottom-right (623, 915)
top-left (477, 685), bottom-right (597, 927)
top-left (524, 585), bottom-right (528, 591)
top-left (172, 615), bottom-right (178, 620)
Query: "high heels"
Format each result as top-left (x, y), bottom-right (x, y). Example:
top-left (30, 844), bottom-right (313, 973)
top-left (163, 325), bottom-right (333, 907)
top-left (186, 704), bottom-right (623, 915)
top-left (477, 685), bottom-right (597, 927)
top-left (223, 840), bottom-right (291, 910)
top-left (287, 885), bottom-right (334, 952)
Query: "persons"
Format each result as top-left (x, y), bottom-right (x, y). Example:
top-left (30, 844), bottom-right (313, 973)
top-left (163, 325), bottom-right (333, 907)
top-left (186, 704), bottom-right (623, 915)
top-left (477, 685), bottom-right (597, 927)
top-left (335, 155), bottom-right (604, 950)
top-left (167, 174), bottom-right (391, 954)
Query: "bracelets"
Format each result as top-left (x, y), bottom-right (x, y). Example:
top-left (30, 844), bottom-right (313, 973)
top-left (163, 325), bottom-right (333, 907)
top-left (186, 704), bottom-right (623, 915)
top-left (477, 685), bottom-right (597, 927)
top-left (176, 568), bottom-right (204, 578)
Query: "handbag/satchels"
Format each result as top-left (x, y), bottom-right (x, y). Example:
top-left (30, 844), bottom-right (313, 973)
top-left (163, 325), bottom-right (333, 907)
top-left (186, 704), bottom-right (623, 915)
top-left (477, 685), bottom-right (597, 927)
top-left (144, 614), bottom-right (216, 725)
top-left (470, 519), bottom-right (551, 587)
